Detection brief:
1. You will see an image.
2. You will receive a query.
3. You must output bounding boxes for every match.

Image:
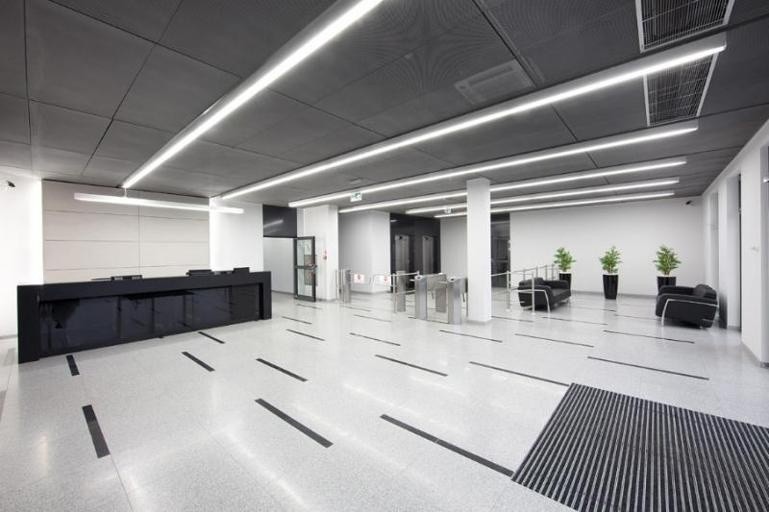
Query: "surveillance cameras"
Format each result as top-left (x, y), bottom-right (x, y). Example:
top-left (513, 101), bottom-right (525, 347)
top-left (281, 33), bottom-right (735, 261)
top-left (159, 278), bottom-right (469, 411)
top-left (685, 200), bottom-right (692, 205)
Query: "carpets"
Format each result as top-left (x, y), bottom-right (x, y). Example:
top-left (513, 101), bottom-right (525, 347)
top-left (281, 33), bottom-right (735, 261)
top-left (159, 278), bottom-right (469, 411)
top-left (509, 384), bottom-right (768, 512)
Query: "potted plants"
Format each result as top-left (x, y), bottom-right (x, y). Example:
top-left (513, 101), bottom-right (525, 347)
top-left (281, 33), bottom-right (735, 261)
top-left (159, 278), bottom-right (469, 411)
top-left (652, 243), bottom-right (682, 292)
top-left (554, 246), bottom-right (575, 296)
top-left (599, 245), bottom-right (622, 301)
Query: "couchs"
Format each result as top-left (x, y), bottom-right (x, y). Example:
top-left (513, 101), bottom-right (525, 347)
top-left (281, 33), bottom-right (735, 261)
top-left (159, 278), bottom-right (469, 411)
top-left (517, 276), bottom-right (571, 311)
top-left (654, 283), bottom-right (717, 330)
